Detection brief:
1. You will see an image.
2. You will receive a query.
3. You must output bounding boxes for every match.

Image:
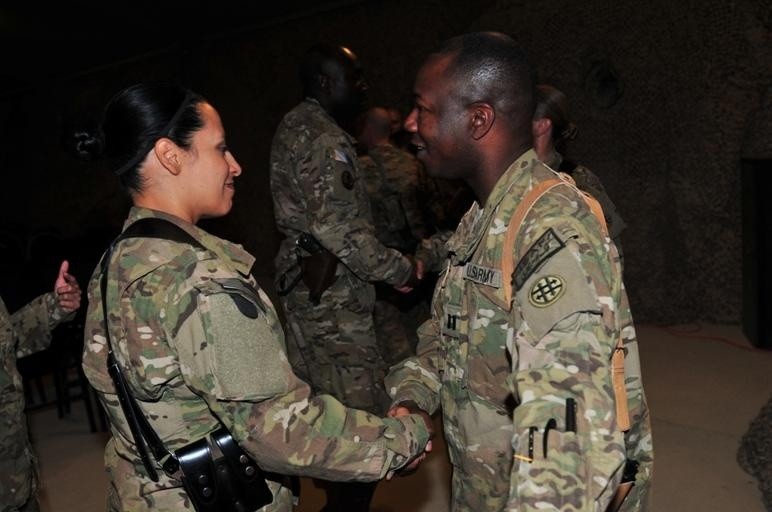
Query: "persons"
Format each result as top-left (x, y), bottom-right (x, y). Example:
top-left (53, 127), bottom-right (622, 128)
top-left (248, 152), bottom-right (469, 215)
top-left (394, 82), bottom-right (623, 323)
top-left (271, 48), bottom-right (423, 512)
top-left (387, 33), bottom-right (653, 512)
top-left (1, 260), bottom-right (82, 510)
top-left (68, 78), bottom-right (433, 511)
top-left (386, 108), bottom-right (447, 236)
top-left (352, 104), bottom-right (441, 372)
